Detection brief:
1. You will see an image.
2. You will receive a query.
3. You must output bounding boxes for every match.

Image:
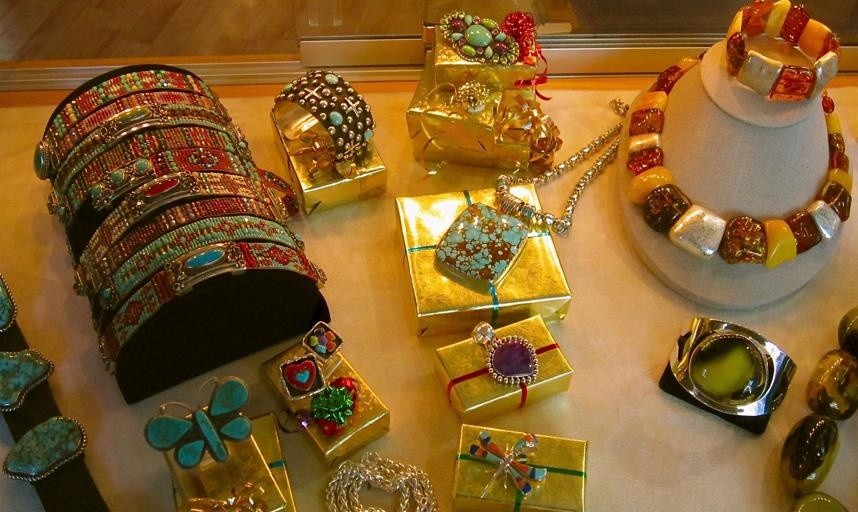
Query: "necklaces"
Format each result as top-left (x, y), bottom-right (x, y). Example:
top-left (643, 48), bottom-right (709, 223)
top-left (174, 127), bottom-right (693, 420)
top-left (434, 99), bottom-right (630, 289)
top-left (627, 51), bottom-right (852, 269)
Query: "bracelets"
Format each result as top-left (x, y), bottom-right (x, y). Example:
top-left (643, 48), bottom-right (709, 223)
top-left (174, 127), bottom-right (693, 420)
top-left (33, 72), bottom-right (220, 179)
top-left (91, 149), bottom-right (300, 217)
top-left (48, 104), bottom-right (244, 216)
top-left (273, 68), bottom-right (377, 180)
top-left (725, 0), bottom-right (838, 104)
top-left (72, 172), bottom-right (288, 297)
top-left (90, 220), bottom-right (306, 335)
top-left (98, 243), bottom-right (326, 378)
top-left (57, 127), bottom-right (254, 228)
top-left (86, 205), bottom-right (287, 303)
top-left (45, 92), bottom-right (232, 180)
